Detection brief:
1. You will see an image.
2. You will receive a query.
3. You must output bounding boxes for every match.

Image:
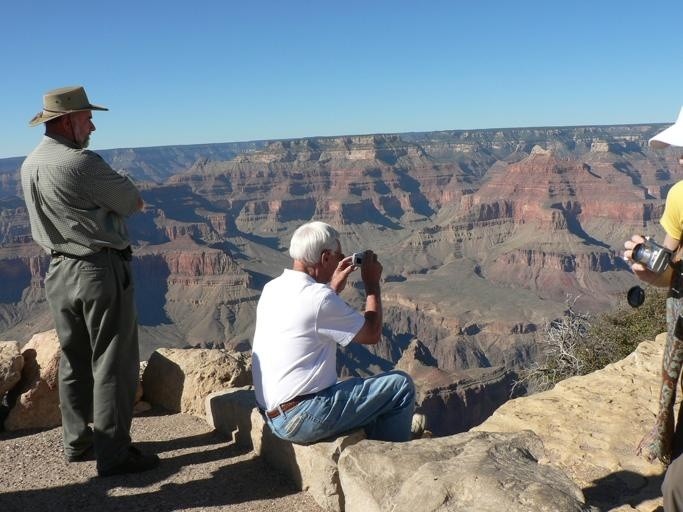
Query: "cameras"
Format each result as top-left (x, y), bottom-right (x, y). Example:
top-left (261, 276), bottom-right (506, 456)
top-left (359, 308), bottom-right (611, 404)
top-left (352, 252), bottom-right (363, 267)
top-left (632, 240), bottom-right (673, 273)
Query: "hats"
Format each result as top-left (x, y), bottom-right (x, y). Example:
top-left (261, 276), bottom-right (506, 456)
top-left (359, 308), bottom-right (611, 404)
top-left (647, 105), bottom-right (683, 150)
top-left (25, 85), bottom-right (108, 129)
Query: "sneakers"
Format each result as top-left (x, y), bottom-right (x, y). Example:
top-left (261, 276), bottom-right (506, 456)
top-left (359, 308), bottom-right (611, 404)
top-left (65, 448), bottom-right (97, 462)
top-left (96, 452), bottom-right (159, 478)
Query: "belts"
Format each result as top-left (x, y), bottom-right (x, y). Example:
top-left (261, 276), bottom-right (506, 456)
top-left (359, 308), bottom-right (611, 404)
top-left (254, 393), bottom-right (310, 419)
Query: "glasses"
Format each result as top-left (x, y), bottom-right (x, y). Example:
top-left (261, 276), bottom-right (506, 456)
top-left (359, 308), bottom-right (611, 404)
top-left (325, 248), bottom-right (345, 262)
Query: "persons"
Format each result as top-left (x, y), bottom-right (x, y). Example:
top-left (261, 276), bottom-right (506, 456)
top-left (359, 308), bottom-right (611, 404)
top-left (247, 219), bottom-right (431, 442)
top-left (623, 103), bottom-right (681, 464)
top-left (17, 87), bottom-right (156, 476)
